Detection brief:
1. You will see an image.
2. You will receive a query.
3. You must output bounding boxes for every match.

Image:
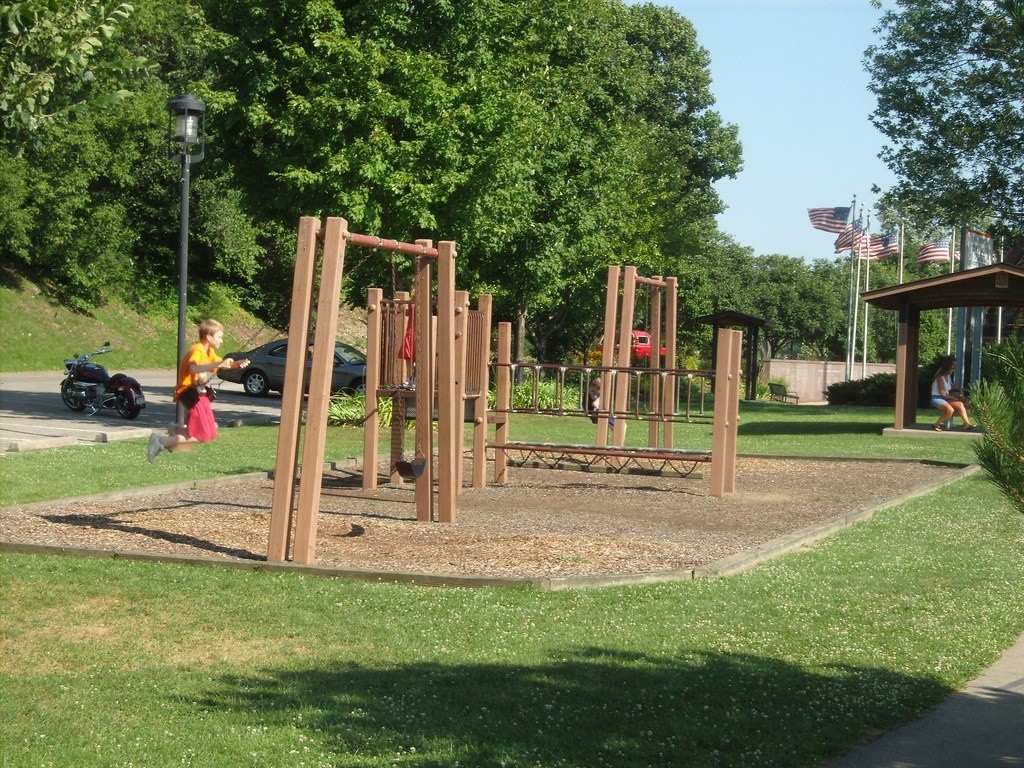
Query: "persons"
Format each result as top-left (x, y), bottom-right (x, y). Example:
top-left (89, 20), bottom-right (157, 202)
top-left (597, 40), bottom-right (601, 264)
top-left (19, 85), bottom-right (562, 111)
top-left (584, 376), bottom-right (627, 435)
top-left (147, 318), bottom-right (251, 464)
top-left (397, 288), bottom-right (420, 390)
top-left (930, 358), bottom-right (976, 432)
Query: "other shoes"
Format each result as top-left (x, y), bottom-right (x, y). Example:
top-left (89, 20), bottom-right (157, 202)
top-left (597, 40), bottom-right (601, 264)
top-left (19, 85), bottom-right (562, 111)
top-left (934, 425), bottom-right (943, 432)
top-left (964, 424), bottom-right (976, 430)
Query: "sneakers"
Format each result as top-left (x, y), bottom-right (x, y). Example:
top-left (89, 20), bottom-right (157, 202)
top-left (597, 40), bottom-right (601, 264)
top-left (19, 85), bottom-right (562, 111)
top-left (166, 421), bottom-right (178, 452)
top-left (148, 433), bottom-right (165, 463)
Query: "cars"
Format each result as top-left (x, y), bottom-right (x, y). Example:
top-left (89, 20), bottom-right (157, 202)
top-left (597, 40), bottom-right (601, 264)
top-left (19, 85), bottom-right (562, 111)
top-left (597, 330), bottom-right (666, 361)
top-left (217, 337), bottom-right (367, 397)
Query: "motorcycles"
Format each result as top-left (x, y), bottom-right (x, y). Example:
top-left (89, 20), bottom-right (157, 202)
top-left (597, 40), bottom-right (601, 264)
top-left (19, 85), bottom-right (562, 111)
top-left (60, 341), bottom-right (146, 419)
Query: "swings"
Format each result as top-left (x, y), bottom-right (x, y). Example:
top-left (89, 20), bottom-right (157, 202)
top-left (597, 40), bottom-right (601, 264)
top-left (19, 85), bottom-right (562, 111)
top-left (178, 244), bottom-right (381, 410)
top-left (390, 251), bottom-right (428, 478)
top-left (643, 284), bottom-right (660, 414)
top-left (585, 282), bottom-right (641, 421)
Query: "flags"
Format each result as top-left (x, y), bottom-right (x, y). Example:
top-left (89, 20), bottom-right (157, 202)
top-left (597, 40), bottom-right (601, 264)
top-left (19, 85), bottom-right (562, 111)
top-left (870, 231), bottom-right (899, 259)
top-left (917, 235), bottom-right (949, 263)
top-left (806, 206), bottom-right (853, 234)
top-left (834, 219), bottom-right (861, 254)
top-left (854, 236), bottom-right (867, 259)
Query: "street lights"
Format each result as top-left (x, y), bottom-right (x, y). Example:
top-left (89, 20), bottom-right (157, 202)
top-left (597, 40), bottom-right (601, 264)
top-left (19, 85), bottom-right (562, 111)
top-left (163, 94), bottom-right (206, 424)
